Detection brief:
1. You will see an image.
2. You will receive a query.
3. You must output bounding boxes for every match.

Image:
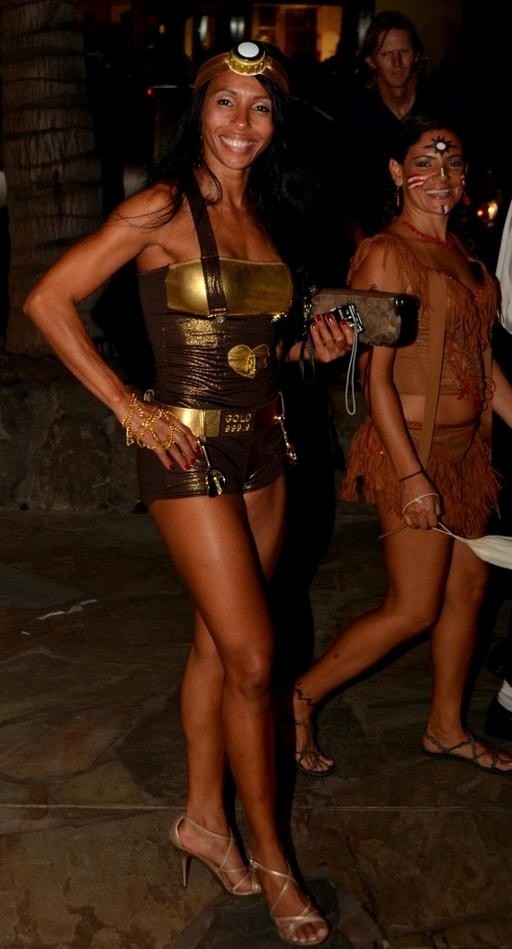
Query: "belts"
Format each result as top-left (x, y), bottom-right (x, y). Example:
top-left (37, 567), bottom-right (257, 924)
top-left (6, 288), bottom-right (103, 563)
top-left (152, 400), bottom-right (289, 440)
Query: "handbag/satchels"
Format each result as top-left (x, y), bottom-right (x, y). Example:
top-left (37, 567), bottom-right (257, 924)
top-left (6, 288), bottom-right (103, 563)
top-left (302, 287), bottom-right (420, 348)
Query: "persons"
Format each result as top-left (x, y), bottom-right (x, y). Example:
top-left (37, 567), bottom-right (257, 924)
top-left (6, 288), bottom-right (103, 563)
top-left (268, 122), bottom-right (510, 782)
top-left (326, 9), bottom-right (458, 289)
top-left (19, 42), bottom-right (358, 946)
top-left (489, 207), bottom-right (511, 339)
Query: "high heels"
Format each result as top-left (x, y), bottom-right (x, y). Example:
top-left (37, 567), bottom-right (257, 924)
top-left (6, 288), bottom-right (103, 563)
top-left (250, 852), bottom-right (329, 944)
top-left (167, 812), bottom-right (261, 895)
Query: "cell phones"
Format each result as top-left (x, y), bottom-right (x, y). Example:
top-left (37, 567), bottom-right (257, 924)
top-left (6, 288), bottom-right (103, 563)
top-left (307, 304), bottom-right (364, 349)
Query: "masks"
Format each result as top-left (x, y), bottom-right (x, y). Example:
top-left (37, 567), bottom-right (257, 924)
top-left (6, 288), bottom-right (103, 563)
top-left (440, 523), bottom-right (512, 573)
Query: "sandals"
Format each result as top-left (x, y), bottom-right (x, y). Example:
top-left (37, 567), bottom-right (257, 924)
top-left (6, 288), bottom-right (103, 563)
top-left (275, 684), bottom-right (336, 775)
top-left (423, 729), bottom-right (510, 774)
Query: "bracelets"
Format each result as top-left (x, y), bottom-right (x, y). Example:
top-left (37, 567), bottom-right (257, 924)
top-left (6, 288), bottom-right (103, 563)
top-left (121, 393), bottom-right (184, 451)
top-left (396, 470), bottom-right (424, 483)
